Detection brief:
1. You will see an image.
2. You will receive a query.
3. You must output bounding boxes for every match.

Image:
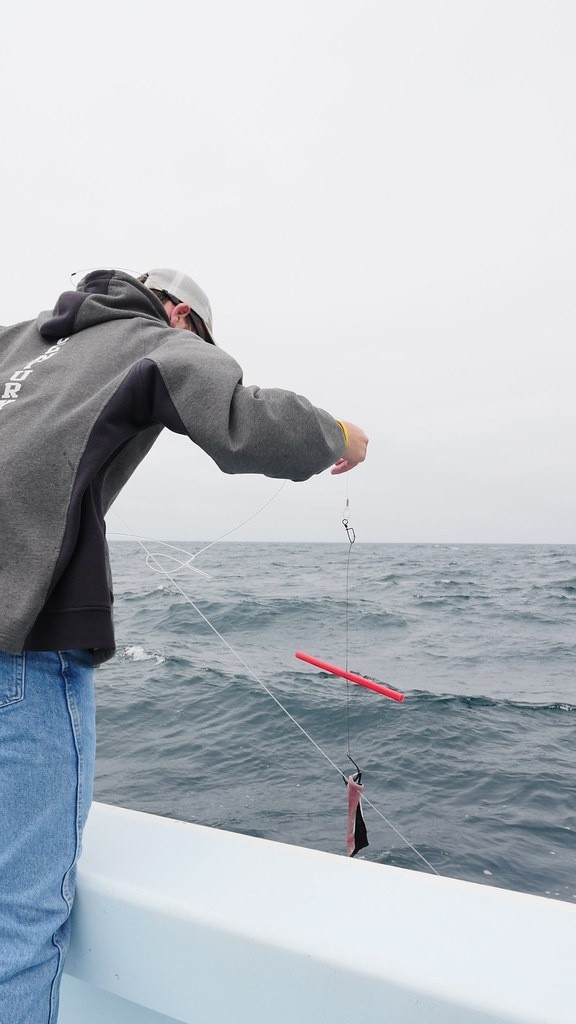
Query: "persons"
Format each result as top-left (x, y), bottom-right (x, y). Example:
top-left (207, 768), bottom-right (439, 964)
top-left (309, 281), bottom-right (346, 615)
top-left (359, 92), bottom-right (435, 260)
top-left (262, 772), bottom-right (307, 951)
top-left (0, 267), bottom-right (369, 1024)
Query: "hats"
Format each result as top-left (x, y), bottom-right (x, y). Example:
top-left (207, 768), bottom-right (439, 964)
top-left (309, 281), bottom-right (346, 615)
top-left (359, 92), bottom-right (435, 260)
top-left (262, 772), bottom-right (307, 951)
top-left (136, 269), bottom-right (218, 347)
